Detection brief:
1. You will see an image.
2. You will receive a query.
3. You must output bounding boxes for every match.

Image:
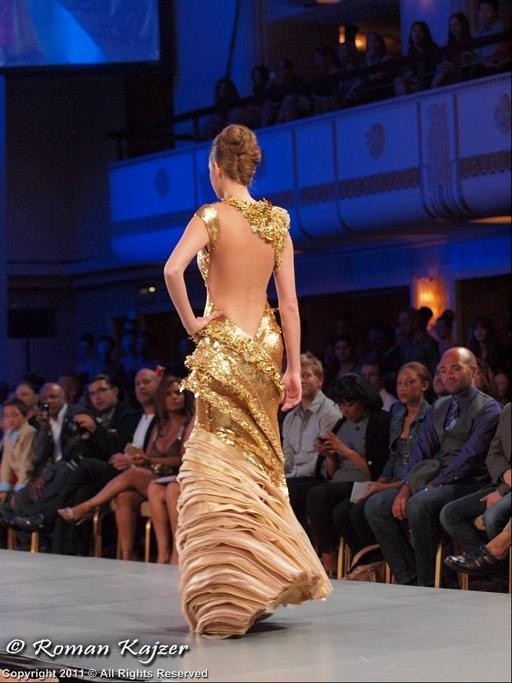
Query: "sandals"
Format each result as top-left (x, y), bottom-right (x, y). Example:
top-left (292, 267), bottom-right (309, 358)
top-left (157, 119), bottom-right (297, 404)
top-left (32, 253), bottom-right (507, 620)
top-left (318, 543), bottom-right (338, 579)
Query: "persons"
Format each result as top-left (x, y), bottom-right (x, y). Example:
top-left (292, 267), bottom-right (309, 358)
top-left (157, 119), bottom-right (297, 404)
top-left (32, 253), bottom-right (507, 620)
top-left (2, 305), bottom-right (511, 596)
top-left (163, 124), bottom-right (336, 640)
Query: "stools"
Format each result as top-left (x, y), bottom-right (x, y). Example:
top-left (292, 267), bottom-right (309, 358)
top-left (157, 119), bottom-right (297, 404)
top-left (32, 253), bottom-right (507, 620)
top-left (1, 481), bottom-right (512, 594)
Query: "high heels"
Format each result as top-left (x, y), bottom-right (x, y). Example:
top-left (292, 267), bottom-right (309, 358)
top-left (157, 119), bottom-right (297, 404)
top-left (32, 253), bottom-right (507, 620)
top-left (56, 506), bottom-right (92, 525)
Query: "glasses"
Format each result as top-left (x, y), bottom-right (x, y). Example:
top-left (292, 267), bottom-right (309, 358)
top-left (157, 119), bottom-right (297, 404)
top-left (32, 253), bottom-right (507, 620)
top-left (88, 386), bottom-right (112, 396)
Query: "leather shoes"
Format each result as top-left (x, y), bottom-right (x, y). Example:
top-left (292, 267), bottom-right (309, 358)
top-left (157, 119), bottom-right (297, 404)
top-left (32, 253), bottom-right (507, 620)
top-left (10, 512), bottom-right (55, 533)
top-left (2, 512), bottom-right (30, 528)
top-left (444, 543), bottom-right (506, 575)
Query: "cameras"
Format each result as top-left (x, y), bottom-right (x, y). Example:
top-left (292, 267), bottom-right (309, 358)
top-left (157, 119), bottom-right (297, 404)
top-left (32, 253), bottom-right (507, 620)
top-left (66, 420), bottom-right (83, 437)
top-left (317, 437), bottom-right (329, 444)
top-left (37, 400), bottom-right (48, 411)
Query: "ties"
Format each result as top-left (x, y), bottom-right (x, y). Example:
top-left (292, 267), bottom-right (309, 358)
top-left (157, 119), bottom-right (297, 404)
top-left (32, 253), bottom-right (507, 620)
top-left (448, 403), bottom-right (458, 425)
top-left (102, 416), bottom-right (110, 429)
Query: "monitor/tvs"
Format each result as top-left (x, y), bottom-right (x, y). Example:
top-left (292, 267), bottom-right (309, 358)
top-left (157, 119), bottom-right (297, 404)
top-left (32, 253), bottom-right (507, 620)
top-left (8, 308), bottom-right (56, 338)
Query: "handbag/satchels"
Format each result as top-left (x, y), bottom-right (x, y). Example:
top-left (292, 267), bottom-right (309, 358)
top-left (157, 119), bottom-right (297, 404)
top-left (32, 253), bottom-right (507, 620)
top-left (345, 543), bottom-right (386, 582)
